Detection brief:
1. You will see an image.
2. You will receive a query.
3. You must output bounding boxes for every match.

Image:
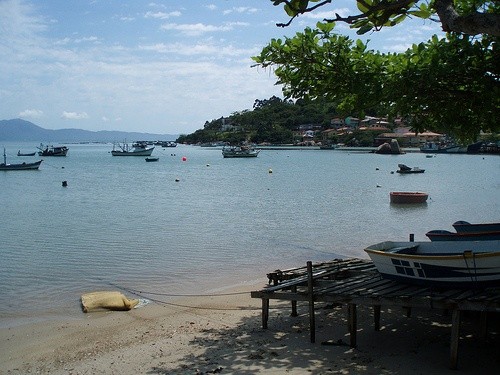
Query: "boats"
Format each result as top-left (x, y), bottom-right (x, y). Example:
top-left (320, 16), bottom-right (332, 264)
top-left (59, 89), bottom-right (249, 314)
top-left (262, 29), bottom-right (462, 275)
top-left (389, 191), bottom-right (428, 203)
top-left (145, 157), bottom-right (159, 162)
top-left (320, 145), bottom-right (335, 150)
top-left (425, 230), bottom-right (500, 242)
top-left (35, 143), bottom-right (69, 156)
top-left (452, 220), bottom-right (500, 232)
top-left (147, 140), bottom-right (177, 147)
top-left (222, 143), bottom-right (261, 158)
top-left (364, 240), bottom-right (500, 285)
top-left (0, 146), bottom-right (44, 170)
top-left (110, 140), bottom-right (155, 157)
top-left (16, 150), bottom-right (36, 156)
top-left (420, 142), bottom-right (441, 153)
